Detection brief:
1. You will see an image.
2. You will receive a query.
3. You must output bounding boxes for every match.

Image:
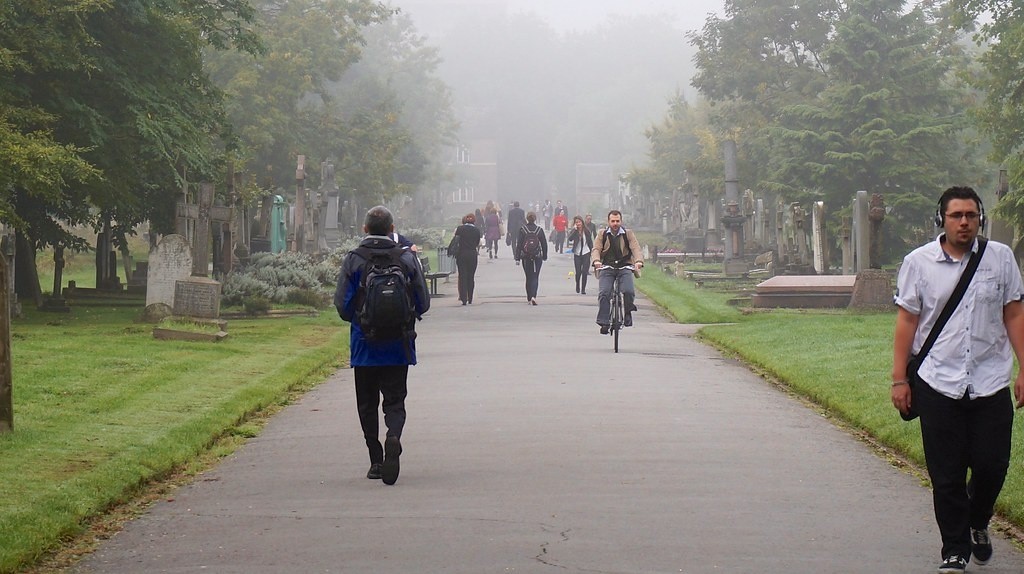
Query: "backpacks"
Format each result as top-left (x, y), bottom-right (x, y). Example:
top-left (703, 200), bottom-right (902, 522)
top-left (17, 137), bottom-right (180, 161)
top-left (348, 247), bottom-right (421, 341)
top-left (520, 224), bottom-right (543, 259)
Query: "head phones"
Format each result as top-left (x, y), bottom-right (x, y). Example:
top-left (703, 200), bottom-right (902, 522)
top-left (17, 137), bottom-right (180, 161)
top-left (935, 199), bottom-right (986, 228)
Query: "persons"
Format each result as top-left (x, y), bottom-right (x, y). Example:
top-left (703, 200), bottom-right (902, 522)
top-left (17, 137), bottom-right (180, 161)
top-left (535, 200), bottom-right (568, 238)
top-left (474, 200), bottom-right (504, 259)
top-left (454, 216), bottom-right (467, 234)
top-left (334, 205), bottom-right (430, 485)
top-left (508, 202), bottom-right (526, 260)
top-left (591, 210), bottom-right (644, 334)
top-left (891, 186), bottom-right (1024, 574)
top-left (389, 233), bottom-right (417, 251)
top-left (553, 209), bottom-right (568, 254)
top-left (516, 212), bottom-right (547, 305)
top-left (455, 214), bottom-right (480, 305)
top-left (568, 215), bottom-right (597, 294)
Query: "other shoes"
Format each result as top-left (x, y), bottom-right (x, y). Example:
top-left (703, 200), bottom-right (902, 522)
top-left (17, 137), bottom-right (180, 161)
top-left (516, 262), bottom-right (519, 265)
top-left (528, 301), bottom-right (531, 305)
top-left (577, 288), bottom-right (580, 293)
top-left (489, 251), bottom-right (493, 258)
top-left (382, 436), bottom-right (403, 485)
top-left (367, 462), bottom-right (382, 479)
top-left (601, 325), bottom-right (610, 334)
top-left (582, 292), bottom-right (586, 294)
top-left (624, 312), bottom-right (632, 326)
top-left (463, 301), bottom-right (466, 305)
top-left (469, 298), bottom-right (472, 303)
top-left (531, 297), bottom-right (537, 306)
top-left (494, 256), bottom-right (497, 258)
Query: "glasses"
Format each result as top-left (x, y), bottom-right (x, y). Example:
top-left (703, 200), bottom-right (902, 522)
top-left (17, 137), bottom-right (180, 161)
top-left (943, 211), bottom-right (981, 222)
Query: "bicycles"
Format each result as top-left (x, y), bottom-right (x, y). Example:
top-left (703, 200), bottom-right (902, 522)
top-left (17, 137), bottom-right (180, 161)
top-left (590, 262), bottom-right (646, 354)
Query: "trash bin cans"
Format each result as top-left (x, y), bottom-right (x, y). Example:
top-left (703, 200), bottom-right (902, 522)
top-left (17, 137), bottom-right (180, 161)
top-left (437, 246), bottom-right (456, 274)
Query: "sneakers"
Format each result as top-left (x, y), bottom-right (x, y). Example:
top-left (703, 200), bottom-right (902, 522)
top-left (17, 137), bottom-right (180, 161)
top-left (970, 526), bottom-right (993, 565)
top-left (937, 555), bottom-right (968, 574)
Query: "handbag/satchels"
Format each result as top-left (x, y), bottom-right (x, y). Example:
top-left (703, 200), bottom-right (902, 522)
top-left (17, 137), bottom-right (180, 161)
top-left (506, 233), bottom-right (512, 246)
top-left (498, 222), bottom-right (506, 236)
top-left (452, 226), bottom-right (463, 258)
top-left (900, 356), bottom-right (919, 422)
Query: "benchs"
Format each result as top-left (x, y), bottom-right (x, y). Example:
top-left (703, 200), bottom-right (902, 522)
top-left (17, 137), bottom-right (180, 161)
top-left (420, 256), bottom-right (451, 298)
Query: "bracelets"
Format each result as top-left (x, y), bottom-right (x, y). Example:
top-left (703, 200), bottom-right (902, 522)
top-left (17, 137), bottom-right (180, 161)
top-left (892, 380), bottom-right (907, 387)
